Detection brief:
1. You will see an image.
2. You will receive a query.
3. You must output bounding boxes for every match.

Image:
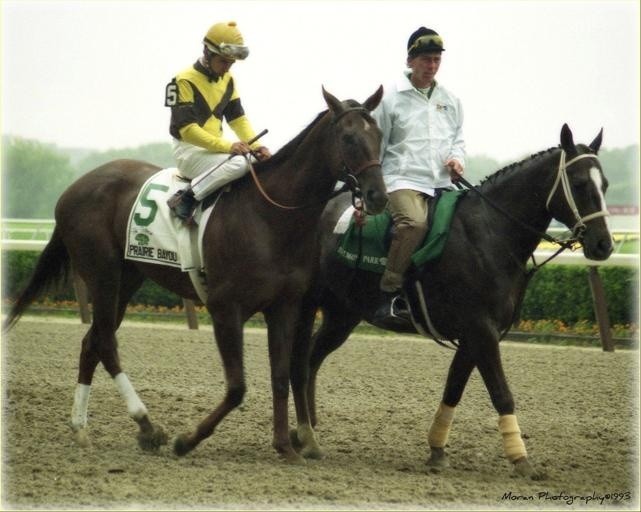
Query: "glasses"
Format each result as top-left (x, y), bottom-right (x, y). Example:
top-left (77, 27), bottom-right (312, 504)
top-left (204, 37), bottom-right (249, 59)
top-left (408, 35), bottom-right (443, 53)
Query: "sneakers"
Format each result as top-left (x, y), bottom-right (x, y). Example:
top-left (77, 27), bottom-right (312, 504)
top-left (372, 303), bottom-right (410, 326)
top-left (167, 190), bottom-right (198, 225)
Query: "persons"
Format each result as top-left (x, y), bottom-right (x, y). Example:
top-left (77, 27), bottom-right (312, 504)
top-left (169, 21), bottom-right (274, 223)
top-left (376, 24), bottom-right (466, 293)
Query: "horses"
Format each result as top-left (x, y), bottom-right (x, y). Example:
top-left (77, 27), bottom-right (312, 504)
top-left (2, 83), bottom-right (388, 467)
top-left (289, 122), bottom-right (615, 478)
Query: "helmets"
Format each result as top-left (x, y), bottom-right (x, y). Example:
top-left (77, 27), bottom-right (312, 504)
top-left (204, 21), bottom-right (243, 60)
top-left (407, 27), bottom-right (445, 56)
